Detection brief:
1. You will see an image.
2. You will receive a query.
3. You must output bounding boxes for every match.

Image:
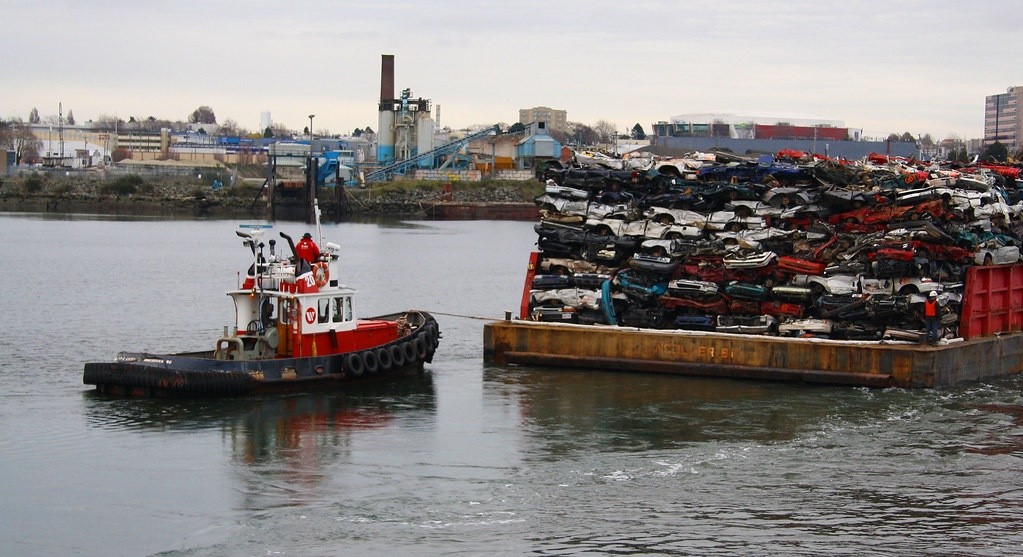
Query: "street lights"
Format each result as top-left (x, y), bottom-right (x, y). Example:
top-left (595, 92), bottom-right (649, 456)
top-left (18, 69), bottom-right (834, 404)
top-left (309, 114), bottom-right (316, 157)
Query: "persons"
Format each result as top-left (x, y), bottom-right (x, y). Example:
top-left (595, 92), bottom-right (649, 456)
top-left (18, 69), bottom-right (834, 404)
top-left (295, 233), bottom-right (320, 263)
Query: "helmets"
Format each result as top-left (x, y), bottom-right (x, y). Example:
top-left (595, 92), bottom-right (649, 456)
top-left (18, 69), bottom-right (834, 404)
top-left (929, 290), bottom-right (937, 296)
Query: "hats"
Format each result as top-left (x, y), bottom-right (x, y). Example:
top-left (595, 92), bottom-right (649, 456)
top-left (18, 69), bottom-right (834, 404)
top-left (303, 233), bottom-right (312, 237)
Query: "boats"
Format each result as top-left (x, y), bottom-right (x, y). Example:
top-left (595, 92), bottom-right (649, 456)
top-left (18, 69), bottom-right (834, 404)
top-left (287, 200), bottom-right (339, 262)
top-left (82, 230), bottom-right (441, 399)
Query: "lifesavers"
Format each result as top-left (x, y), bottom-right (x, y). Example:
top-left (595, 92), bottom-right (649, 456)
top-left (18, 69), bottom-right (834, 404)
top-left (387, 345), bottom-right (404, 368)
top-left (411, 337), bottom-right (426, 360)
top-left (424, 320), bottom-right (438, 347)
top-left (399, 342), bottom-right (416, 364)
top-left (343, 353), bottom-right (364, 376)
top-left (313, 261), bottom-right (330, 287)
top-left (360, 351), bottom-right (378, 373)
top-left (375, 348), bottom-right (392, 371)
top-left (417, 331), bottom-right (433, 354)
